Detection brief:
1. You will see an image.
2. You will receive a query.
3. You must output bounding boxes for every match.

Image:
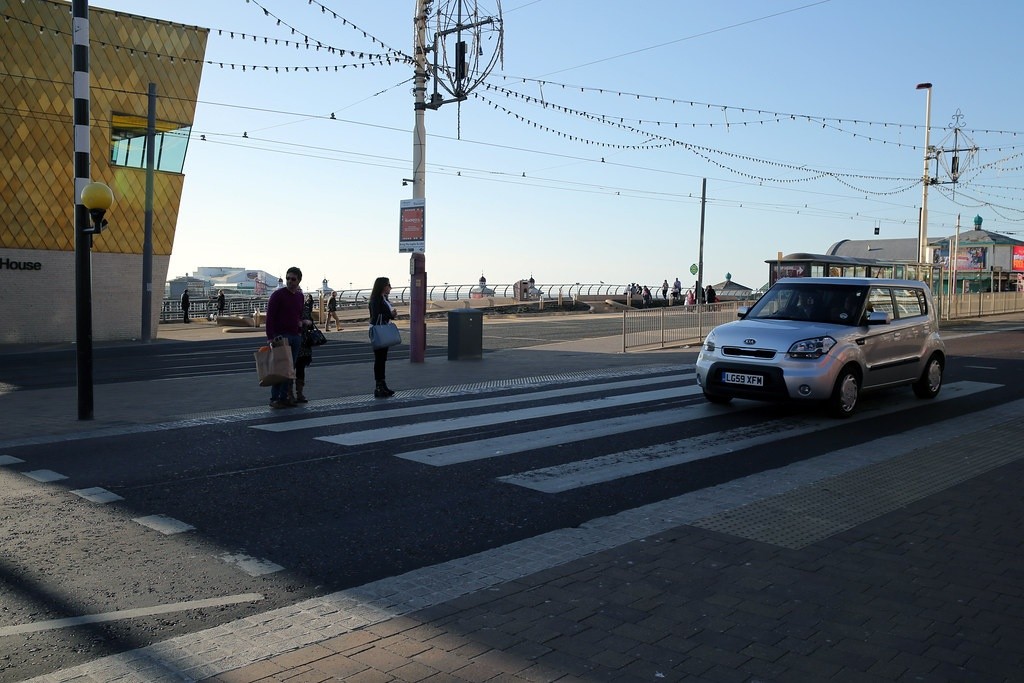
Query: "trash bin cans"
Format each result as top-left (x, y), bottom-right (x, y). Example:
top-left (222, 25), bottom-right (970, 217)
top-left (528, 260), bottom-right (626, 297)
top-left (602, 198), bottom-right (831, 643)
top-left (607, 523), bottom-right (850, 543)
top-left (447, 308), bottom-right (484, 360)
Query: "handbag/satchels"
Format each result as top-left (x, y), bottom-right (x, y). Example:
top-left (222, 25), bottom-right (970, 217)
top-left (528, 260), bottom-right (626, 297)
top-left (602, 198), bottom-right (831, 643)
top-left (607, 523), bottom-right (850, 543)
top-left (302, 321), bottom-right (327, 347)
top-left (368, 313), bottom-right (401, 349)
top-left (254, 335), bottom-right (296, 387)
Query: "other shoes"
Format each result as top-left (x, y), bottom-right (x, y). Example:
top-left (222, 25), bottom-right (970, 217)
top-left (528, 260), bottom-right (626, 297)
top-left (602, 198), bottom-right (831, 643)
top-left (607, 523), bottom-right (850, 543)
top-left (296, 397), bottom-right (308, 403)
top-left (280, 399), bottom-right (298, 408)
top-left (288, 394), bottom-right (297, 403)
top-left (270, 400), bottom-right (286, 409)
top-left (326, 330), bottom-right (330, 331)
top-left (338, 329), bottom-right (344, 331)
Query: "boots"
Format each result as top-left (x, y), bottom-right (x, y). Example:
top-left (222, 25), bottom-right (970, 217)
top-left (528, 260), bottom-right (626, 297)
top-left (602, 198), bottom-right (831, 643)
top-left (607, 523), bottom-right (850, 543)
top-left (374, 379), bottom-right (395, 397)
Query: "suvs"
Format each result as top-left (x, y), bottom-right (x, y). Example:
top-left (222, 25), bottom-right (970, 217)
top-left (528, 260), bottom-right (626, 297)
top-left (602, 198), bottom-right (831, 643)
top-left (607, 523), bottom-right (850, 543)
top-left (695, 276), bottom-right (950, 420)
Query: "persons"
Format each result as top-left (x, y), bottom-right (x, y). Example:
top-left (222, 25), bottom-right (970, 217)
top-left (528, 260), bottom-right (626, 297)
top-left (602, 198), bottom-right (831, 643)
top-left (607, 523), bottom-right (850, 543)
top-left (305, 295), bottom-right (313, 313)
top-left (707, 285), bottom-right (715, 303)
top-left (368, 277), bottom-right (397, 398)
top-left (636, 284), bottom-right (652, 308)
top-left (325, 291), bottom-right (344, 332)
top-left (798, 291), bottom-right (814, 317)
top-left (266, 267), bottom-right (313, 404)
top-left (624, 284), bottom-right (631, 295)
top-left (181, 289), bottom-right (190, 323)
top-left (217, 289), bottom-right (225, 316)
top-left (688, 290), bottom-right (694, 305)
top-left (673, 277), bottom-right (681, 300)
top-left (662, 280), bottom-right (669, 300)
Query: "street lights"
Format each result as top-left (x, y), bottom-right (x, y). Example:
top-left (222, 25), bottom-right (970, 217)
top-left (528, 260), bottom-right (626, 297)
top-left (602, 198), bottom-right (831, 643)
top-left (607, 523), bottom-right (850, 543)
top-left (915, 81), bottom-right (933, 280)
top-left (70, 0), bottom-right (116, 424)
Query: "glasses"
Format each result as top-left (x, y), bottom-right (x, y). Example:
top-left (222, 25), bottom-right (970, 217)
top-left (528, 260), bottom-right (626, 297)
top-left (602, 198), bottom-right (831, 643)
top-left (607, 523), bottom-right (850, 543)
top-left (286, 277), bottom-right (298, 281)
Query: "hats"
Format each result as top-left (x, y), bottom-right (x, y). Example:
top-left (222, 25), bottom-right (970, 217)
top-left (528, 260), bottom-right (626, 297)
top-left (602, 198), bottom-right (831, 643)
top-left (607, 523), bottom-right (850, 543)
top-left (184, 290), bottom-right (189, 292)
